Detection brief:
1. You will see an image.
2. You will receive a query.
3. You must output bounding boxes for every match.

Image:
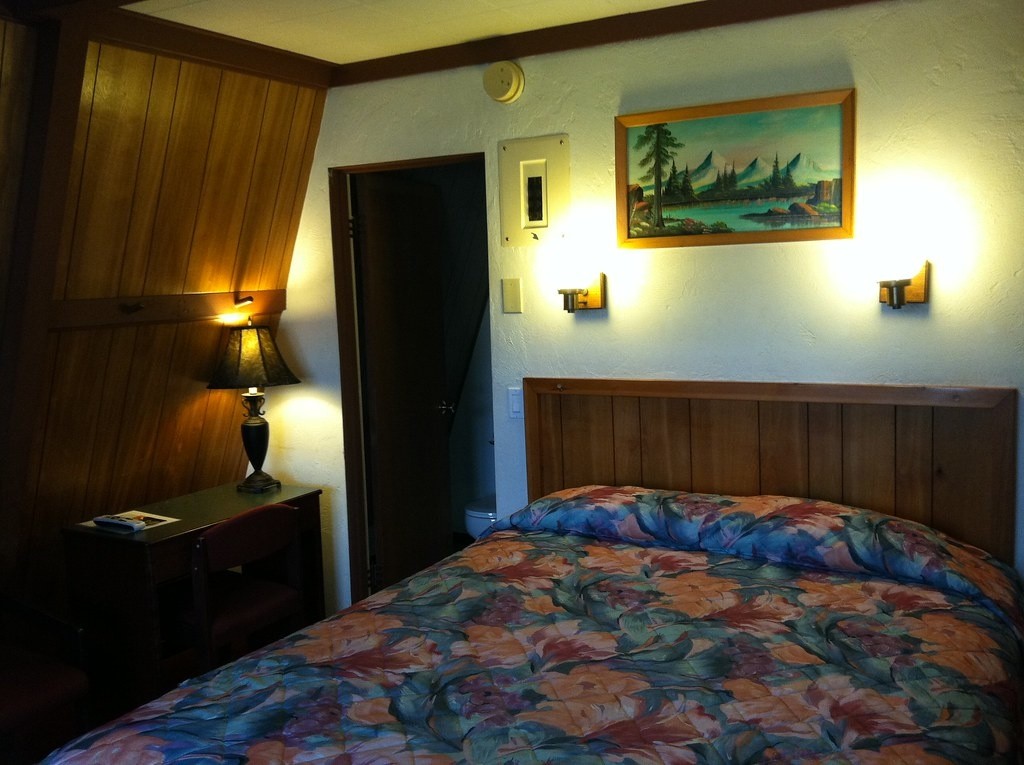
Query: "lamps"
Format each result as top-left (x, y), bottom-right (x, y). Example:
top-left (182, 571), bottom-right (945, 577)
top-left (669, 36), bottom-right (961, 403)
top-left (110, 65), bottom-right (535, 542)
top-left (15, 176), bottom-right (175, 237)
top-left (546, 255), bottom-right (604, 313)
top-left (204, 317), bottom-right (301, 494)
top-left (865, 244), bottom-right (930, 310)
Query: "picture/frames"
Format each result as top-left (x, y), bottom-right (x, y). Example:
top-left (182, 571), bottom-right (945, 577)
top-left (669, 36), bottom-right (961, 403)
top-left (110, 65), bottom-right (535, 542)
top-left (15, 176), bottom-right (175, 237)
top-left (613, 88), bottom-right (858, 250)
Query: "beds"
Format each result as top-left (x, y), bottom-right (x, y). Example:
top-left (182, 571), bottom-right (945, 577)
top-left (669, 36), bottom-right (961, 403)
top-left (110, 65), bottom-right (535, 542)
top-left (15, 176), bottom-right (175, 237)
top-left (45, 378), bottom-right (1024, 765)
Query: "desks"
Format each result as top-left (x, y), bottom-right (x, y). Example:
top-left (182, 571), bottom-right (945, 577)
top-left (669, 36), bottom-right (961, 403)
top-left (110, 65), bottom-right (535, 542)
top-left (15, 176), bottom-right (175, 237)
top-left (60, 483), bottom-right (325, 703)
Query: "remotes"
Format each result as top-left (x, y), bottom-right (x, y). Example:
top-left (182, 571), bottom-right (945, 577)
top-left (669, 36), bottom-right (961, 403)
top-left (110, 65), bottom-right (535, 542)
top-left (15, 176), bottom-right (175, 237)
top-left (93, 515), bottom-right (146, 533)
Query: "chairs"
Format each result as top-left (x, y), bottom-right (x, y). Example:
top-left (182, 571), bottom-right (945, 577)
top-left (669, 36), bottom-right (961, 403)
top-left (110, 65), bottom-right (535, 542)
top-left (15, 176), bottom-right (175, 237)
top-left (165, 504), bottom-right (304, 676)
top-left (0, 586), bottom-right (89, 765)
